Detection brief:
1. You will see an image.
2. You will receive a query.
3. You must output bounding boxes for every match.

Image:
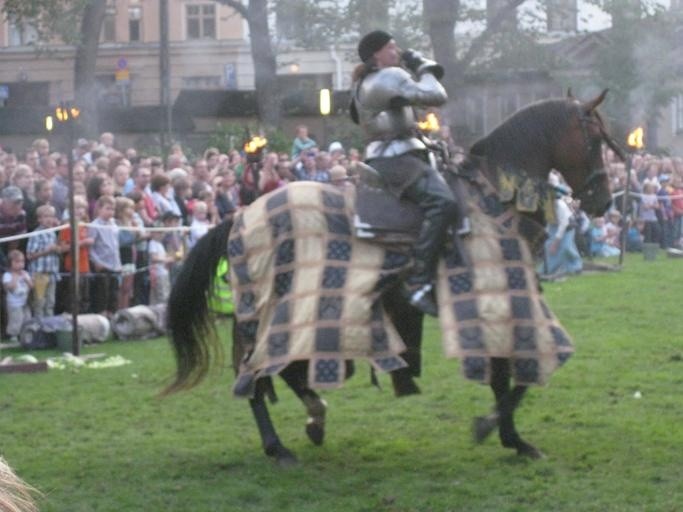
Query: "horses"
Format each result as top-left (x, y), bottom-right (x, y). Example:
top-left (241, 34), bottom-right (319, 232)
top-left (155, 86), bottom-right (625, 470)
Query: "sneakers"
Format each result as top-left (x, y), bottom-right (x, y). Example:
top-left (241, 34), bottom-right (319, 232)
top-left (399, 280), bottom-right (438, 316)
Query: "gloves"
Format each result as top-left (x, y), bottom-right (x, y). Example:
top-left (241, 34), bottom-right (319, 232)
top-left (403, 49), bottom-right (443, 78)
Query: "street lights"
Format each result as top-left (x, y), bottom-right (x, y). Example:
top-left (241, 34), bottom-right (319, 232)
top-left (319, 87), bottom-right (332, 148)
top-left (619, 127), bottom-right (644, 264)
top-left (243, 121), bottom-right (269, 196)
top-left (46, 100), bottom-right (83, 357)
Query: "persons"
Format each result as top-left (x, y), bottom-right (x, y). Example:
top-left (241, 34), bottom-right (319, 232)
top-left (351, 30), bottom-right (458, 315)
top-left (0, 138), bottom-right (69, 341)
top-left (54, 127), bottom-right (358, 318)
top-left (536, 147), bottom-right (683, 281)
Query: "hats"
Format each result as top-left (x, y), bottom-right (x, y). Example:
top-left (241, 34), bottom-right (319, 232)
top-left (359, 31), bottom-right (392, 61)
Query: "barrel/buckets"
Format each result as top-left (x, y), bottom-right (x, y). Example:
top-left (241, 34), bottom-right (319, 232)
top-left (642, 243), bottom-right (659, 261)
